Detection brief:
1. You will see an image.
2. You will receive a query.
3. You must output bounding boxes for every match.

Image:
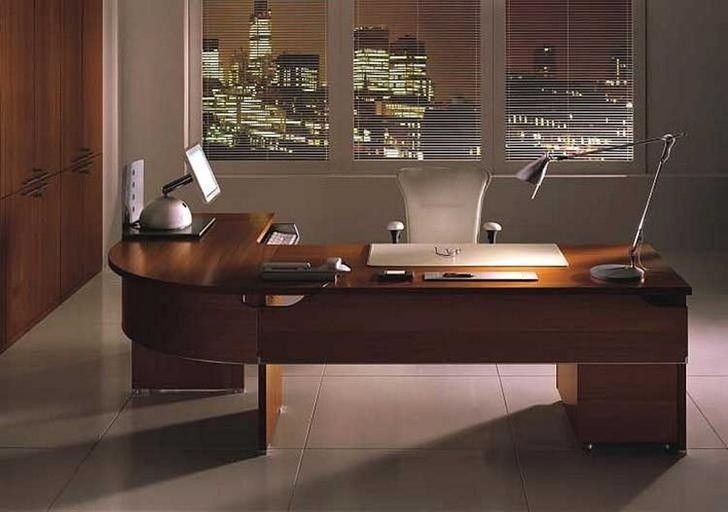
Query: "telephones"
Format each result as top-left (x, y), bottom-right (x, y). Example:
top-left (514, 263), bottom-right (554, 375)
top-left (262, 257), bottom-right (341, 282)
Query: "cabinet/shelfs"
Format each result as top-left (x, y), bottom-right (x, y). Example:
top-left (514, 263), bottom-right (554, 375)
top-left (1, 1), bottom-right (105, 354)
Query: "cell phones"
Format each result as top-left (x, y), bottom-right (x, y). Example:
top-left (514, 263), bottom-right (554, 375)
top-left (376, 270), bottom-right (413, 282)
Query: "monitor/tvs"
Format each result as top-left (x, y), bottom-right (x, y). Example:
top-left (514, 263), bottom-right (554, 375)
top-left (181, 142), bottom-right (221, 203)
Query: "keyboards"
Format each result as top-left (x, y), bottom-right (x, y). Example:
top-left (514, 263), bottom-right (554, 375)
top-left (265, 230), bottom-right (297, 245)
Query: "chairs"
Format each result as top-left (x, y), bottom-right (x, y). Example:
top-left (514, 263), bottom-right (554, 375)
top-left (385, 161), bottom-right (502, 244)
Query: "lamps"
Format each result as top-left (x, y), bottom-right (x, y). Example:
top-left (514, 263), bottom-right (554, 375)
top-left (514, 135), bottom-right (678, 288)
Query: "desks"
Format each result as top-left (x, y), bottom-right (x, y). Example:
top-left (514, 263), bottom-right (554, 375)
top-left (106, 207), bottom-right (695, 458)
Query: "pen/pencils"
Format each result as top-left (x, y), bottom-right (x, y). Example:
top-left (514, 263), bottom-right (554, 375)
top-left (444, 274), bottom-right (474, 277)
